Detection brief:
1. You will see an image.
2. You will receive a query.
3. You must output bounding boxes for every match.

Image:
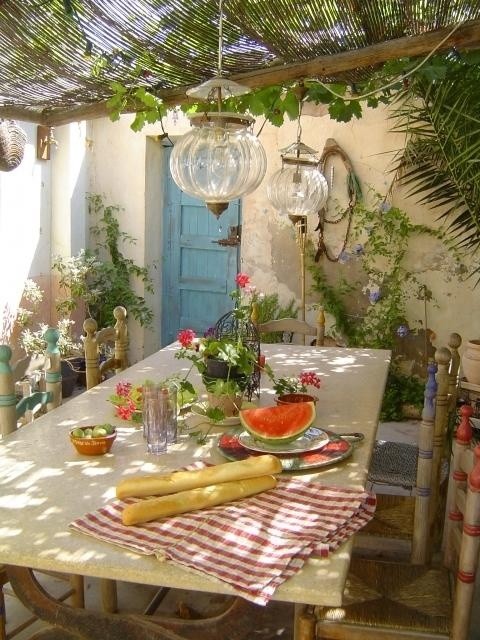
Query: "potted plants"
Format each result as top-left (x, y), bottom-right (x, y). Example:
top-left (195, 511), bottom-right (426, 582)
top-left (110, 304), bottom-right (321, 419)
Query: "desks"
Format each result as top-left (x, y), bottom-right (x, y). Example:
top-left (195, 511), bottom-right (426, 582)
top-left (2, 338), bottom-right (392, 640)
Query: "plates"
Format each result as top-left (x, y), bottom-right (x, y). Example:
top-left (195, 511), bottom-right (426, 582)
top-left (236, 428), bottom-right (330, 455)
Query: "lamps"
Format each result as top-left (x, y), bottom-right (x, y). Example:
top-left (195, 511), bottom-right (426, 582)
top-left (169, 0), bottom-right (330, 229)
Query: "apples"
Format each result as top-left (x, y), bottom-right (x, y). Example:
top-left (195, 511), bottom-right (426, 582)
top-left (73, 423), bottom-right (114, 438)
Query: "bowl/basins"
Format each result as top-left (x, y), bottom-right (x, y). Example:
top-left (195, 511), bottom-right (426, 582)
top-left (67, 425), bottom-right (117, 456)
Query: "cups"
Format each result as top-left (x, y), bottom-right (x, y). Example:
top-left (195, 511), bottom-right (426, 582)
top-left (141, 383), bottom-right (178, 456)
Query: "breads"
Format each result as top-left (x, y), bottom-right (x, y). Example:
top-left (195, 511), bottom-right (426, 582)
top-left (121, 475), bottom-right (277, 525)
top-left (116, 454), bottom-right (283, 503)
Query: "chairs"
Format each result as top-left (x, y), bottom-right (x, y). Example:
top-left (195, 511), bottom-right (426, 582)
top-left (249, 304), bottom-right (325, 347)
top-left (292, 404), bottom-right (480, 640)
top-left (340, 366), bottom-right (438, 561)
top-left (83, 306), bottom-right (132, 390)
top-left (364, 331), bottom-right (462, 497)
top-left (2, 327), bottom-right (84, 637)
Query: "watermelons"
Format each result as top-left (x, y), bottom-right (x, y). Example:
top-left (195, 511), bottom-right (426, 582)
top-left (238, 401), bottom-right (316, 446)
